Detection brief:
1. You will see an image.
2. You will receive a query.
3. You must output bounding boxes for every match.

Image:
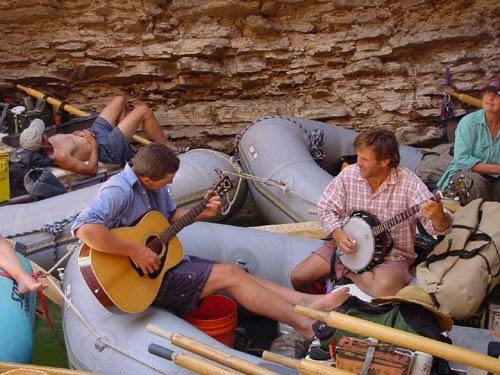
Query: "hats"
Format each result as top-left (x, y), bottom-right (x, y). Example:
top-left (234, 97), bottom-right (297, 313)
top-left (480, 76), bottom-right (499, 95)
top-left (370, 286), bottom-right (454, 333)
top-left (19, 119), bottom-right (44, 151)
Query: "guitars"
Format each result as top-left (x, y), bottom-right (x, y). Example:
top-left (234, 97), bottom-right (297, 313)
top-left (75, 168), bottom-right (234, 317)
top-left (335, 172), bottom-right (474, 274)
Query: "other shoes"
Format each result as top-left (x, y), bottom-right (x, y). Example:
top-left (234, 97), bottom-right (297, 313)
top-left (295, 337), bottom-right (335, 366)
top-left (312, 319), bottom-right (337, 346)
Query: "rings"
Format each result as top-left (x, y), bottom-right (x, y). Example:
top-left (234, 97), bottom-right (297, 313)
top-left (352, 240), bottom-right (356, 243)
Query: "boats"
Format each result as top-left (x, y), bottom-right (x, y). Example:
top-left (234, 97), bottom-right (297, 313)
top-left (0, 249), bottom-right (36, 364)
top-left (236, 115), bottom-right (424, 222)
top-left (63, 221), bottom-right (499, 374)
top-left (1, 148), bottom-right (247, 273)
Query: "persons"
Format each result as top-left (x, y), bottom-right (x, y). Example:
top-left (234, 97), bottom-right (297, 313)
top-left (0, 234), bottom-right (48, 295)
top-left (20, 95), bottom-right (190, 176)
top-left (290, 127), bottom-right (454, 297)
top-left (71, 144), bottom-right (350, 341)
top-left (437, 82), bottom-right (500, 207)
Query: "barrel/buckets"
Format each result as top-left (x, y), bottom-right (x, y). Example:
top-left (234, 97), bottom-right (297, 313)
top-left (186, 293), bottom-right (237, 349)
